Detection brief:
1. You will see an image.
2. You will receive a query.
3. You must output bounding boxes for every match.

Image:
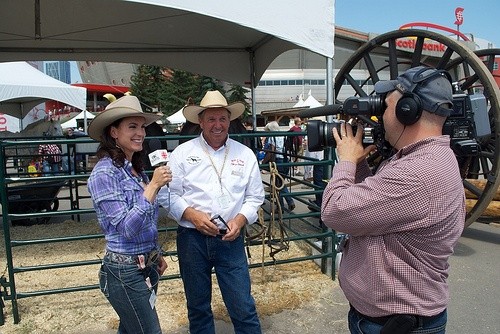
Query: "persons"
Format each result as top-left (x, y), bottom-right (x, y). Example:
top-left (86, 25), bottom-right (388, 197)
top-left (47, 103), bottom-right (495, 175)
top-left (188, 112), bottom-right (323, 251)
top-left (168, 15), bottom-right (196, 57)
top-left (22, 129), bottom-right (82, 187)
top-left (87, 96), bottom-right (173, 334)
top-left (321, 66), bottom-right (466, 334)
top-left (157, 90), bottom-right (265, 334)
top-left (264, 121), bottom-right (296, 214)
top-left (246, 116), bottom-right (323, 189)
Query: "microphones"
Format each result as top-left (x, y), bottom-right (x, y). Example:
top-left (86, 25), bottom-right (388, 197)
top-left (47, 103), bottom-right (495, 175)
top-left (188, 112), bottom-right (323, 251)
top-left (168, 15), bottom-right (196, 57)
top-left (149, 139), bottom-right (169, 169)
top-left (299, 103), bottom-right (345, 117)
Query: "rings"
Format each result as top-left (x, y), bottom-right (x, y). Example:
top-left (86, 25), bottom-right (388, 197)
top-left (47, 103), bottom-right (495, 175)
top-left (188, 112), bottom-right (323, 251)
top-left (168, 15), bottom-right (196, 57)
top-left (233, 237), bottom-right (236, 239)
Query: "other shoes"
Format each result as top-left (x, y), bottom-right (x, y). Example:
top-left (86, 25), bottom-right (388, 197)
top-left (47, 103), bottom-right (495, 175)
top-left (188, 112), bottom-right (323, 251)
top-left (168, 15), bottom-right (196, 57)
top-left (271, 209), bottom-right (284, 214)
top-left (288, 204), bottom-right (295, 210)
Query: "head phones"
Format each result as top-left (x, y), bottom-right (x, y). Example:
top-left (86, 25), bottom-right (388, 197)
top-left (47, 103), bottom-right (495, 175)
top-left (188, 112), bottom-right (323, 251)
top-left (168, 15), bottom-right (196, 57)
top-left (396, 67), bottom-right (456, 125)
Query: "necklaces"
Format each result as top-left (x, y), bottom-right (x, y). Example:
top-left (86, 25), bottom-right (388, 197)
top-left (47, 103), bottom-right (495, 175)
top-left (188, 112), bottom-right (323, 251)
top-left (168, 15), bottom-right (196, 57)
top-left (131, 172), bottom-right (143, 182)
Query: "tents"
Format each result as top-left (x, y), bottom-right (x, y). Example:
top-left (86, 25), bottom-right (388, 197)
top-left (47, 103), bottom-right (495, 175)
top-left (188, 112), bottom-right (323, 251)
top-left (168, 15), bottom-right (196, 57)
top-left (0, 0), bottom-right (336, 277)
top-left (0, 61), bottom-right (326, 136)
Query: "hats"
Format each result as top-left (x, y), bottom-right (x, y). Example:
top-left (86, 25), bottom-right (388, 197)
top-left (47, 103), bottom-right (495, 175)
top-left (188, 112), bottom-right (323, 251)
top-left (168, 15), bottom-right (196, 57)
top-left (182, 90), bottom-right (246, 124)
top-left (87, 91), bottom-right (165, 142)
top-left (374, 65), bottom-right (454, 117)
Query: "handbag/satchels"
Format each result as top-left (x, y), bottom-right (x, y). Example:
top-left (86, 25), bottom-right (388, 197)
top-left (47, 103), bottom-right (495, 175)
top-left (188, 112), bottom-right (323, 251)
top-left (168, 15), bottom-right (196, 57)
top-left (262, 152), bottom-right (276, 172)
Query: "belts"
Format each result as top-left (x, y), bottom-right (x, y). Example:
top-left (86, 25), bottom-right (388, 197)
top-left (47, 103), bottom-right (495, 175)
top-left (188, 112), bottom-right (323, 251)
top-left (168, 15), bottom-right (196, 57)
top-left (349, 302), bottom-right (446, 330)
top-left (106, 251), bottom-right (139, 264)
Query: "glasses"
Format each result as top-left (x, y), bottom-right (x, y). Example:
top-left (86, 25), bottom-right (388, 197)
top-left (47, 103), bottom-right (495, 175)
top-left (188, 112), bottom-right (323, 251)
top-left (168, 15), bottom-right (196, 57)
top-left (210, 214), bottom-right (231, 231)
